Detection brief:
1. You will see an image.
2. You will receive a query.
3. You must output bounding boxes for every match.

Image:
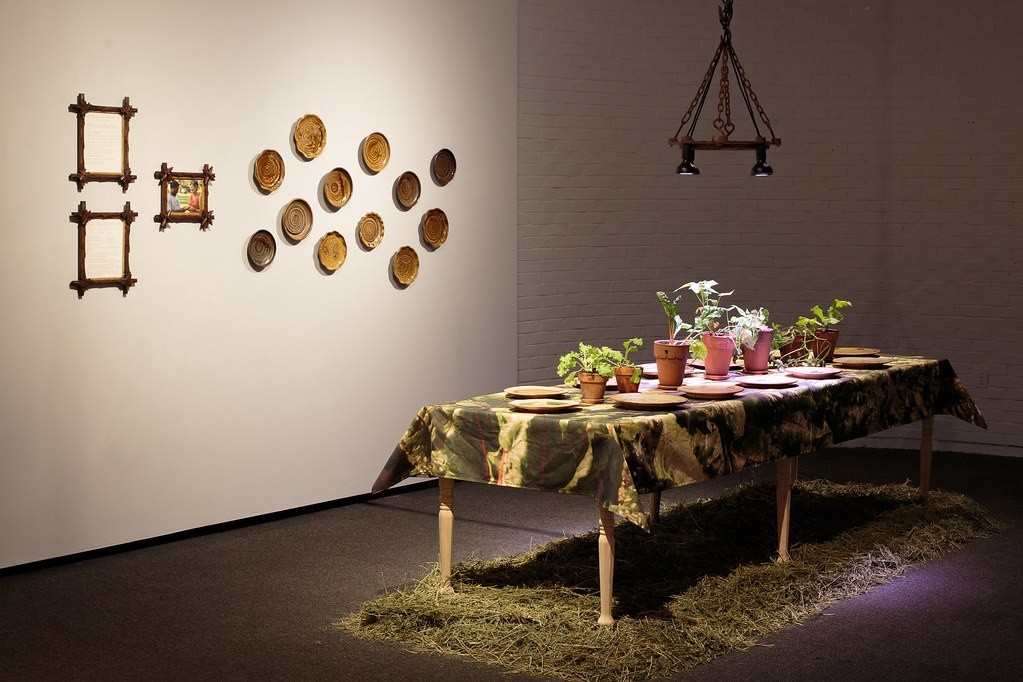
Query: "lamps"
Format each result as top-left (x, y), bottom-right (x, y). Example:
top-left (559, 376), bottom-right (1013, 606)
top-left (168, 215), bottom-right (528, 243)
top-left (668, 0), bottom-right (781, 176)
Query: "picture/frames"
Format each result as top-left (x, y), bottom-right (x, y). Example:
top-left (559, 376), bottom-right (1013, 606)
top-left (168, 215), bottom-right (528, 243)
top-left (154, 162), bottom-right (216, 231)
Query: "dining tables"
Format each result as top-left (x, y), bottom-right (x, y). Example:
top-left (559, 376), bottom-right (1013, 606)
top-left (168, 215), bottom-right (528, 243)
top-left (372, 356), bottom-right (989, 626)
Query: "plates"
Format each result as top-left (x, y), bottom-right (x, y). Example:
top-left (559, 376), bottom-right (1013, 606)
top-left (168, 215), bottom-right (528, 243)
top-left (294, 113), bottom-right (326, 160)
top-left (358, 212), bottom-right (385, 248)
top-left (509, 399), bottom-right (579, 412)
top-left (396, 171), bottom-right (421, 210)
top-left (253, 150), bottom-right (285, 192)
top-left (432, 148), bottom-right (457, 186)
top-left (282, 198), bottom-right (313, 241)
top-left (422, 209), bottom-right (449, 249)
top-left (505, 386), bottom-right (568, 398)
top-left (572, 347), bottom-right (892, 408)
top-left (324, 167), bottom-right (353, 207)
top-left (319, 231), bottom-right (347, 271)
top-left (248, 229), bottom-right (277, 268)
top-left (393, 246), bottom-right (419, 286)
top-left (362, 132), bottom-right (390, 173)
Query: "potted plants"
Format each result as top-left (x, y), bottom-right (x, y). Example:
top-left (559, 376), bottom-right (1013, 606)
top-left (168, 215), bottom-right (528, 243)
top-left (654, 281), bottom-right (851, 388)
top-left (562, 337), bottom-right (645, 402)
